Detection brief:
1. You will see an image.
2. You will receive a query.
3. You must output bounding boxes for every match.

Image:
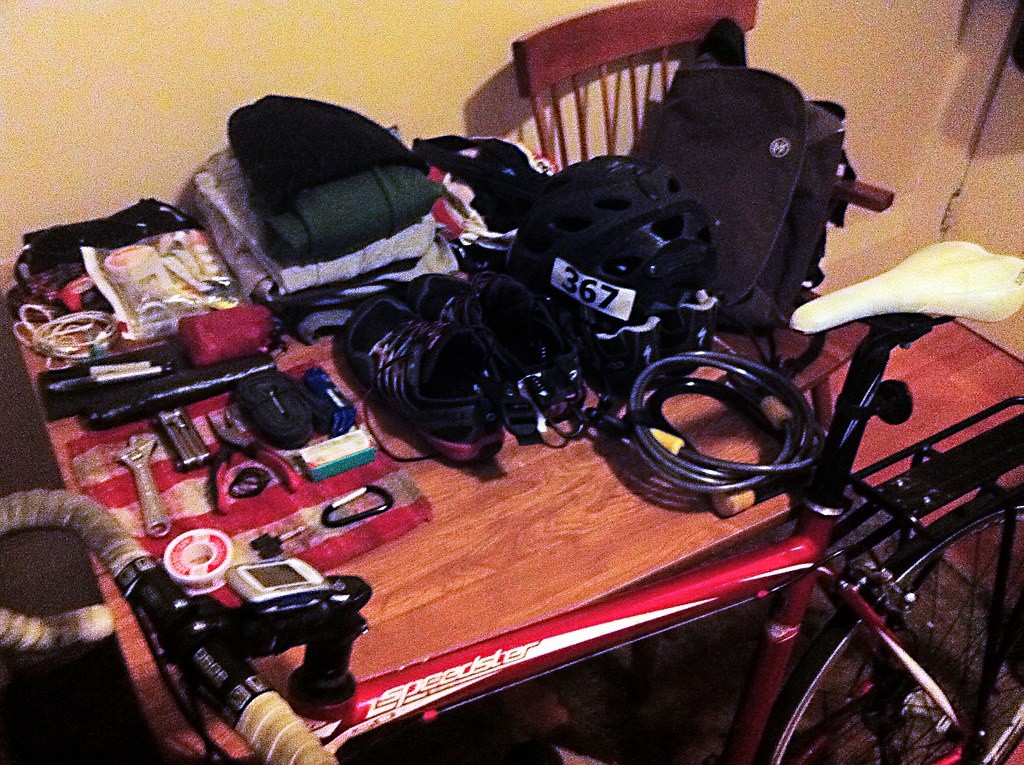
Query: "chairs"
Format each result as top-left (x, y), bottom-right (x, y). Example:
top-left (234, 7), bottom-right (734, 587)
top-left (512, 0), bottom-right (894, 434)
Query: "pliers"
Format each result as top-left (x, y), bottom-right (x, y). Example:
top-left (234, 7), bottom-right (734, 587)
top-left (205, 409), bottom-right (300, 515)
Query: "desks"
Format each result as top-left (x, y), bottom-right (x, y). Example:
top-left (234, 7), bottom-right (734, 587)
top-left (15, 321), bottom-right (789, 752)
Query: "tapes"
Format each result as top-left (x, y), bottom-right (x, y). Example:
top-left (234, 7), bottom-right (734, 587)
top-left (163, 528), bottom-right (234, 597)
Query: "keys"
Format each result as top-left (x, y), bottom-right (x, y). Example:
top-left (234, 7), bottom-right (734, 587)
top-left (249, 524), bottom-right (307, 559)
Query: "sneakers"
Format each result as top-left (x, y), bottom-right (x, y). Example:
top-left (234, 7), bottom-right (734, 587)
top-left (331, 271), bottom-right (587, 467)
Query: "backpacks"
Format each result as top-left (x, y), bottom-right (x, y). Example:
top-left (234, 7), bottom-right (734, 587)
top-left (631, 18), bottom-right (860, 339)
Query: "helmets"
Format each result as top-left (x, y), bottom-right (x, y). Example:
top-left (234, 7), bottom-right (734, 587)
top-left (508, 152), bottom-right (723, 401)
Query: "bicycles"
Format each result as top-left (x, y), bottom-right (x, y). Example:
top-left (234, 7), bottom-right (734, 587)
top-left (0, 240), bottom-right (1024, 765)
